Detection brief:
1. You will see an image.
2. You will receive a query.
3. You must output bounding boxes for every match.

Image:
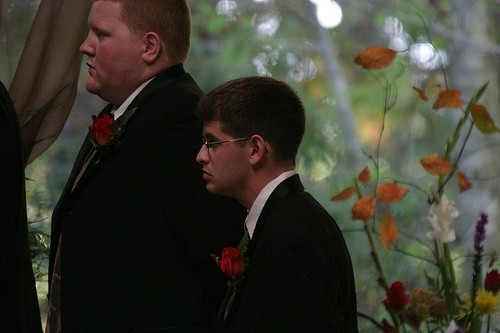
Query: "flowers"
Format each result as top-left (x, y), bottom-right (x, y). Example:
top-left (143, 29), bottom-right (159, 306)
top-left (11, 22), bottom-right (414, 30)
top-left (330, 13), bottom-right (500, 333)
top-left (83, 105), bottom-right (138, 167)
top-left (209, 231), bottom-right (249, 320)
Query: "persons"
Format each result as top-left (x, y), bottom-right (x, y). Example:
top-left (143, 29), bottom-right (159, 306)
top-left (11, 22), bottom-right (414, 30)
top-left (196, 77), bottom-right (358, 333)
top-left (0, 80), bottom-right (42, 333)
top-left (45, 0), bottom-right (248, 333)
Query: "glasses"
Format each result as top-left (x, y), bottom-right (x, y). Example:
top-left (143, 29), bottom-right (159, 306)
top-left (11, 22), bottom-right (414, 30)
top-left (201, 136), bottom-right (268, 153)
